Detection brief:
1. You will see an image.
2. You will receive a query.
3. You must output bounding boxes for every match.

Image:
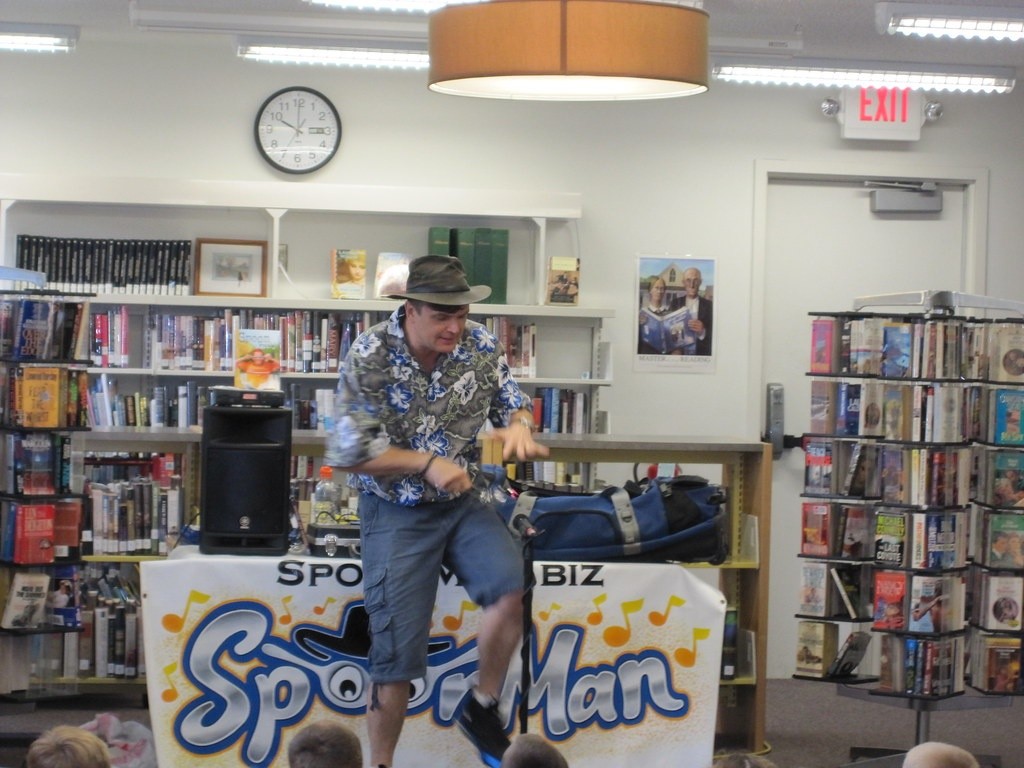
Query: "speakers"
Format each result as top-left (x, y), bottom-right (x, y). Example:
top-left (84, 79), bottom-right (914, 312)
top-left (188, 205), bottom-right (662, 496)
top-left (199, 405), bottom-right (292, 558)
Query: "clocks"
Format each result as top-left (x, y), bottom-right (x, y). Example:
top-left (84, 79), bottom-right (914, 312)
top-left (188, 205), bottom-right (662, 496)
top-left (255, 85), bottom-right (342, 175)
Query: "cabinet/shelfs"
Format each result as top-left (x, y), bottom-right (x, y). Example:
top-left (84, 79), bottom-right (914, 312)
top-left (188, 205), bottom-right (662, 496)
top-left (1, 289), bottom-right (772, 756)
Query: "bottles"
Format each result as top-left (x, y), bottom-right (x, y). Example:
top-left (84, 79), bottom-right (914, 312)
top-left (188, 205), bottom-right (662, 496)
top-left (314, 466), bottom-right (337, 526)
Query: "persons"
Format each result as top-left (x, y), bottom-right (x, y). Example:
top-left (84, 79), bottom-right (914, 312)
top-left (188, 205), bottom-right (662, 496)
top-left (26, 725), bottom-right (111, 768)
top-left (902, 741), bottom-right (980, 768)
top-left (714, 750), bottom-right (774, 768)
top-left (329, 256), bottom-right (551, 768)
top-left (288, 720), bottom-right (363, 768)
top-left (502, 733), bottom-right (569, 768)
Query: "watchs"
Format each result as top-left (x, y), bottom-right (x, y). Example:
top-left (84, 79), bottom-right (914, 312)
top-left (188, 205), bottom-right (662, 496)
top-left (512, 415), bottom-right (539, 433)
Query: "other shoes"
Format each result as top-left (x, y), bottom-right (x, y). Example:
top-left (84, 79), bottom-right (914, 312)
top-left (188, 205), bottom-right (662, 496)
top-left (456, 698), bottom-right (511, 761)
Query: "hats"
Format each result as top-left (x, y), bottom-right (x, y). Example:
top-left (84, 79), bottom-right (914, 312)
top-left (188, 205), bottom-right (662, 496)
top-left (383, 254), bottom-right (492, 307)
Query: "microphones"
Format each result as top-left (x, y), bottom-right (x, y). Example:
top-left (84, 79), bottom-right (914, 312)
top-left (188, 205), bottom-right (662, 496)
top-left (513, 513), bottom-right (536, 539)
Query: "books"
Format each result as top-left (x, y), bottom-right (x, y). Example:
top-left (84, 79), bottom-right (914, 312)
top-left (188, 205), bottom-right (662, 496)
top-left (0, 227), bottom-right (760, 681)
top-left (792, 311), bottom-right (1024, 702)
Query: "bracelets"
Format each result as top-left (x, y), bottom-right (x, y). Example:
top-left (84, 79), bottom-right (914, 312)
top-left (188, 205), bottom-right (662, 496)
top-left (417, 450), bottom-right (442, 478)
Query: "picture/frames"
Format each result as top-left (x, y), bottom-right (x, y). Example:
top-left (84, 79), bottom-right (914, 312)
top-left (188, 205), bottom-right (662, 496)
top-left (194, 237), bottom-right (265, 296)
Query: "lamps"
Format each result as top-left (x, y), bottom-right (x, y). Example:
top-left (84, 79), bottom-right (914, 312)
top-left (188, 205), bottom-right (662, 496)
top-left (822, 82), bottom-right (944, 138)
top-left (874, 1), bottom-right (1024, 40)
top-left (0, 21), bottom-right (79, 53)
top-left (430, 0), bottom-right (709, 103)
top-left (707, 51), bottom-right (1018, 94)
top-left (239, 38), bottom-right (430, 71)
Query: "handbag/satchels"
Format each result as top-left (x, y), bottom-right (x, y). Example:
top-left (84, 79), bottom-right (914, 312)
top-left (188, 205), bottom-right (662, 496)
top-left (511, 475), bottom-right (732, 564)
